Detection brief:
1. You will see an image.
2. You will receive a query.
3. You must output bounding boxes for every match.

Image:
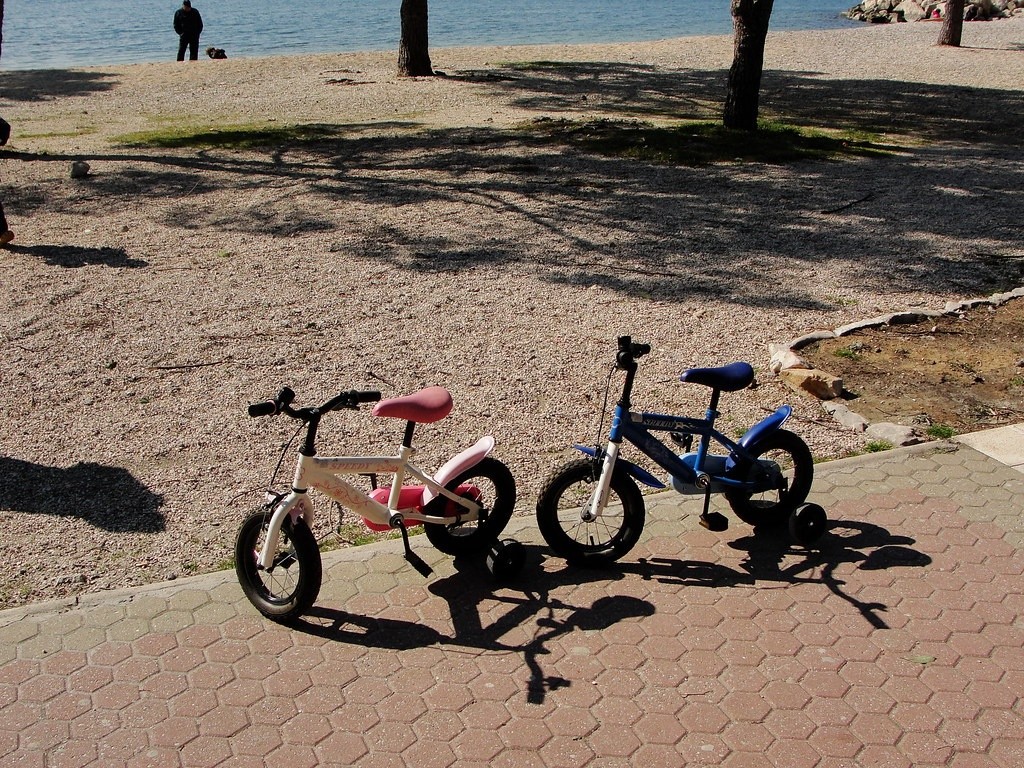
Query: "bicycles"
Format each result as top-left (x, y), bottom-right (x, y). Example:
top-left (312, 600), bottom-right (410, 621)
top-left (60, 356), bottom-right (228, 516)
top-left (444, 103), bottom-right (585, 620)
top-left (533, 336), bottom-right (830, 571)
top-left (231, 385), bottom-right (526, 627)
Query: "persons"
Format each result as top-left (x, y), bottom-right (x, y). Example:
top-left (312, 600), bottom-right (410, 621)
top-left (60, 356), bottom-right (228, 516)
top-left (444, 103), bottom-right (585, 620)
top-left (932, 9), bottom-right (941, 18)
top-left (205, 46), bottom-right (228, 60)
top-left (0, 116), bottom-right (14, 249)
top-left (173, 0), bottom-right (204, 61)
